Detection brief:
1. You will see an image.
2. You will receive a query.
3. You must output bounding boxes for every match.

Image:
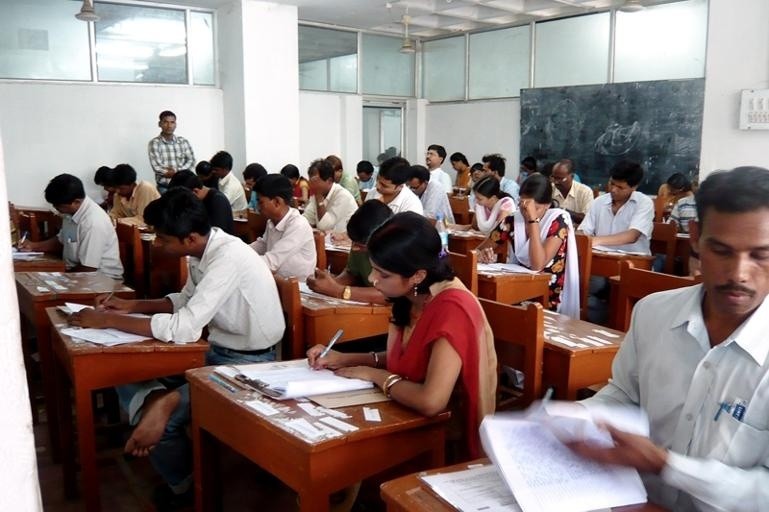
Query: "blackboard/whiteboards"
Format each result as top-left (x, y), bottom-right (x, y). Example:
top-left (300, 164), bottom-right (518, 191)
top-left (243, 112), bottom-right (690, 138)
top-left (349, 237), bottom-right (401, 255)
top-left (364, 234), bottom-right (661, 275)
top-left (520, 77), bottom-right (705, 195)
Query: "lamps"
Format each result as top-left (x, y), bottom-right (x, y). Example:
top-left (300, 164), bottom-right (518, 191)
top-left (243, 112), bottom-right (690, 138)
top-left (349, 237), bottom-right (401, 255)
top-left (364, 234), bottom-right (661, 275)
top-left (397, 15), bottom-right (417, 55)
top-left (75, 1), bottom-right (99, 22)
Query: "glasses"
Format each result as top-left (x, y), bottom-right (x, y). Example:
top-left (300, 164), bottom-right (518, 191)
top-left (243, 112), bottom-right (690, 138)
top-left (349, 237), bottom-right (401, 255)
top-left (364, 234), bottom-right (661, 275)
top-left (409, 183), bottom-right (424, 192)
top-left (548, 173), bottom-right (570, 184)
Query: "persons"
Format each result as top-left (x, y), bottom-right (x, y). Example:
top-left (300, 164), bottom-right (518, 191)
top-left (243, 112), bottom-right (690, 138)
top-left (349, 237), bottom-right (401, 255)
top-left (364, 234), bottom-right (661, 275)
top-left (557, 165), bottom-right (769, 511)
top-left (66, 190), bottom-right (289, 504)
top-left (307, 212), bottom-right (501, 511)
top-left (92, 142), bottom-right (699, 365)
top-left (147, 111), bottom-right (197, 194)
top-left (17, 172), bottom-right (124, 290)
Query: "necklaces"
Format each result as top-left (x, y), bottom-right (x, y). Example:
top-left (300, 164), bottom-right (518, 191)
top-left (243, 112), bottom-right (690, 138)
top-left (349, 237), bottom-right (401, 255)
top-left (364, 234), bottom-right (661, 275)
top-left (400, 294), bottom-right (433, 341)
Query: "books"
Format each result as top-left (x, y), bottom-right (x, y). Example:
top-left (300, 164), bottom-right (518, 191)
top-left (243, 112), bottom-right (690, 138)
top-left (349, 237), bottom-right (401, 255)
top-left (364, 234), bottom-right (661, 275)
top-left (213, 352), bottom-right (375, 404)
top-left (56, 302), bottom-right (155, 350)
top-left (11, 245), bottom-right (47, 265)
top-left (421, 394), bottom-right (651, 511)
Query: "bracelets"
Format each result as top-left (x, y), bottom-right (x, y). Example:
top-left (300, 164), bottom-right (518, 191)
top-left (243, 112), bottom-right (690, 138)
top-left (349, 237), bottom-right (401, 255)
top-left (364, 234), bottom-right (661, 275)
top-left (381, 374), bottom-right (408, 399)
top-left (371, 349), bottom-right (382, 368)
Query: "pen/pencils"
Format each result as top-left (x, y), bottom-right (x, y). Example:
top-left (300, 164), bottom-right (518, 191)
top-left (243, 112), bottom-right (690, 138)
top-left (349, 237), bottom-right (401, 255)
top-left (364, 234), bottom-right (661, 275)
top-left (99, 291), bottom-right (115, 308)
top-left (309, 328), bottom-right (344, 371)
top-left (540, 387), bottom-right (554, 411)
top-left (328, 264), bottom-right (330, 273)
top-left (209, 374), bottom-right (238, 393)
top-left (18, 231), bottom-right (28, 252)
